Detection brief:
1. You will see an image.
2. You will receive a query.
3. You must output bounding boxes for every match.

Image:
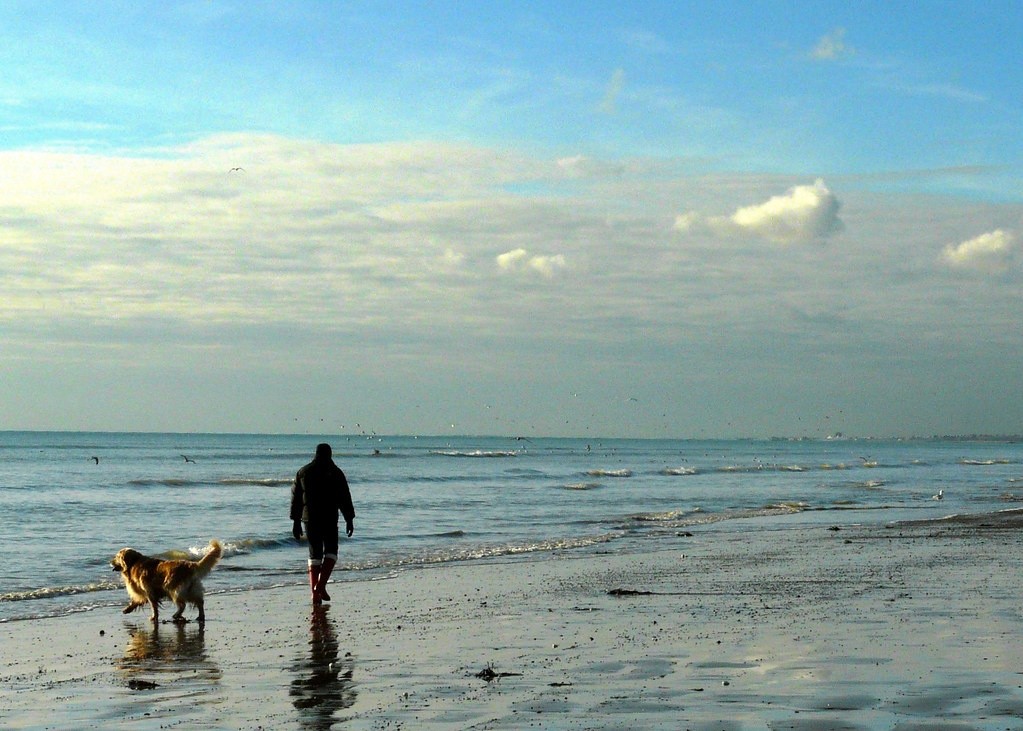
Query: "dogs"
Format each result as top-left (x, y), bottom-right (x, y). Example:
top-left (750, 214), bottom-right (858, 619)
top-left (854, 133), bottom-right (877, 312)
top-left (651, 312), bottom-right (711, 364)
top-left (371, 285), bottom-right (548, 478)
top-left (109, 540), bottom-right (224, 622)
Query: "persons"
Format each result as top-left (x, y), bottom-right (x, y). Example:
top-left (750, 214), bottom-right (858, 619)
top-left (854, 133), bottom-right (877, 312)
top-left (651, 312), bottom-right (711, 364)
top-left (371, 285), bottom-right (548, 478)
top-left (290, 443), bottom-right (355, 604)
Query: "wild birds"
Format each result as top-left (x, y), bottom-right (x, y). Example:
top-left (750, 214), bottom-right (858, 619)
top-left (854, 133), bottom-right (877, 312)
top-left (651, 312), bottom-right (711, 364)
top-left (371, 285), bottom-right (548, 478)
top-left (293, 398), bottom-right (868, 471)
top-left (181, 455), bottom-right (195, 464)
top-left (228, 167), bottom-right (244, 173)
top-left (91, 456), bottom-right (99, 464)
top-left (932, 490), bottom-right (944, 501)
